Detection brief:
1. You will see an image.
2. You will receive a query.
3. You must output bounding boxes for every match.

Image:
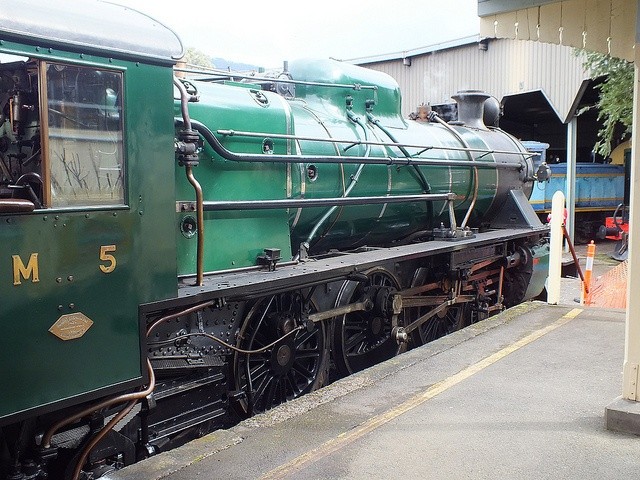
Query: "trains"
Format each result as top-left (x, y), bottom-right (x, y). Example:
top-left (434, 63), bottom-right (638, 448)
top-left (0, 0), bottom-right (579, 478)
top-left (519, 141), bottom-right (632, 244)
